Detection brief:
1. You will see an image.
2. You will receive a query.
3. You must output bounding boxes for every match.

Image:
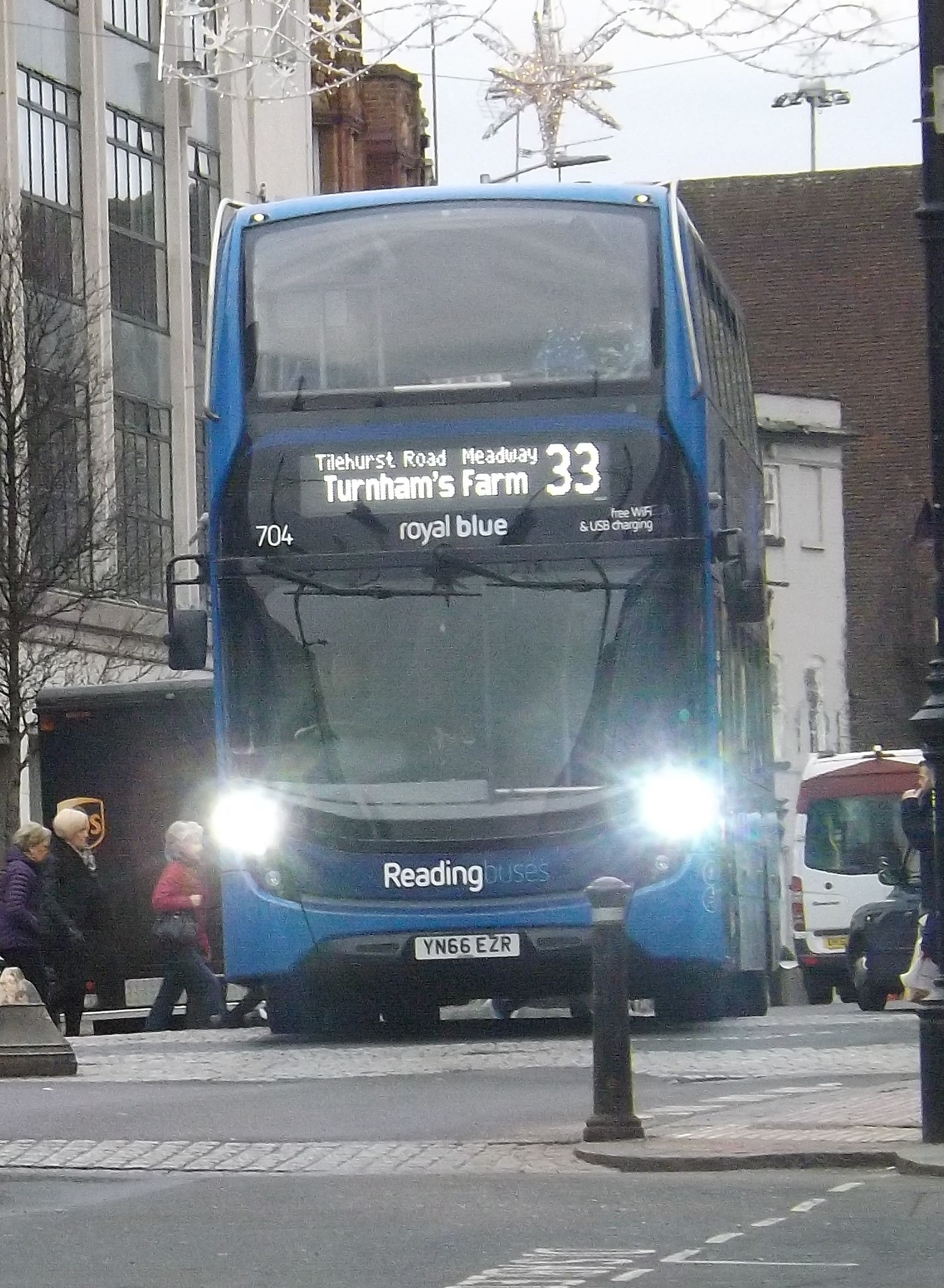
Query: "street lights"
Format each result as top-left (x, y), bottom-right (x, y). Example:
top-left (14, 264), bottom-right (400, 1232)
top-left (771, 75), bottom-right (852, 172)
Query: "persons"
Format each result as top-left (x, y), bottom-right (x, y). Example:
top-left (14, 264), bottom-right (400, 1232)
top-left (485, 999), bottom-right (593, 1018)
top-left (901, 761), bottom-right (940, 907)
top-left (0, 823), bottom-right (50, 1011)
top-left (141, 821), bottom-right (222, 1033)
top-left (45, 808), bottom-right (98, 1036)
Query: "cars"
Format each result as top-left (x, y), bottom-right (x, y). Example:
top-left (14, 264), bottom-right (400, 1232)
top-left (846, 842), bottom-right (921, 1013)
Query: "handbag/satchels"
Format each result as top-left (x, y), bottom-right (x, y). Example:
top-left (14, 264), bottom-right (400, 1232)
top-left (151, 910), bottom-right (198, 949)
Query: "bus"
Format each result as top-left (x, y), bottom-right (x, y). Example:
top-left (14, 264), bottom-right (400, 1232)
top-left (784, 741), bottom-right (927, 1005)
top-left (162, 181), bottom-right (780, 1038)
